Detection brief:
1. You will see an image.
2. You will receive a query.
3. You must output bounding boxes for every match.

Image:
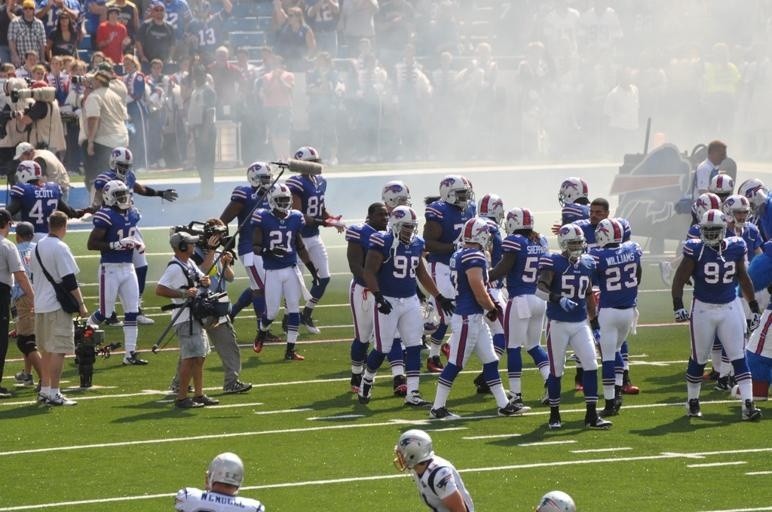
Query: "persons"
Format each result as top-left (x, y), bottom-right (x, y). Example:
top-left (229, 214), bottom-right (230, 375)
top-left (157, 219), bottom-right (252, 407)
top-left (176, 449), bottom-right (264, 512)
top-left (0, 147), bottom-right (179, 405)
top-left (2, 1), bottom-right (771, 219)
top-left (537, 486), bottom-right (581, 512)
top-left (391, 428), bottom-right (477, 511)
top-left (346, 176), bottom-right (642, 430)
top-left (221, 148), bottom-right (346, 360)
top-left (673, 178), bottom-right (771, 419)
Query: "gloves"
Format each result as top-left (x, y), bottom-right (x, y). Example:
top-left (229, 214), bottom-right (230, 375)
top-left (486, 306), bottom-right (498, 321)
top-left (110, 236), bottom-right (135, 251)
top-left (435, 293), bottom-right (457, 316)
top-left (262, 246), bottom-right (289, 259)
top-left (323, 214), bottom-right (347, 234)
top-left (374, 292), bottom-right (393, 315)
top-left (495, 301), bottom-right (503, 315)
top-left (134, 239), bottom-right (146, 254)
top-left (673, 307), bottom-right (689, 322)
top-left (162, 188), bottom-right (177, 202)
top-left (592, 329), bottom-right (601, 344)
top-left (749, 311), bottom-right (761, 332)
top-left (312, 268), bottom-right (322, 289)
top-left (558, 295), bottom-right (579, 313)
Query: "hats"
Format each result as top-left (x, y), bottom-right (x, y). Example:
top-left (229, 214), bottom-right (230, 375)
top-left (205, 217), bottom-right (228, 233)
top-left (0, 209), bottom-right (14, 224)
top-left (12, 142), bottom-right (33, 160)
top-left (169, 231), bottom-right (200, 247)
top-left (22, 0), bottom-right (35, 9)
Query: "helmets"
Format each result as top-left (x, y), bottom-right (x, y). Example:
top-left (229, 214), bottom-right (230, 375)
top-left (558, 176), bottom-right (589, 207)
top-left (101, 179), bottom-right (131, 210)
top-left (16, 159), bottom-right (41, 184)
top-left (393, 428), bottom-right (435, 472)
top-left (246, 161), bottom-right (275, 189)
top-left (439, 175), bottom-right (472, 210)
top-left (594, 216), bottom-right (622, 249)
top-left (616, 217), bottom-right (631, 242)
top-left (695, 192), bottom-right (723, 220)
top-left (461, 218), bottom-right (495, 250)
top-left (558, 223), bottom-right (587, 259)
top-left (204, 451), bottom-right (244, 487)
top-left (699, 208), bottom-right (728, 248)
top-left (387, 205), bottom-right (419, 245)
top-left (536, 489), bottom-right (577, 512)
top-left (381, 179), bottom-right (413, 208)
top-left (267, 182), bottom-right (293, 215)
top-left (710, 173), bottom-right (735, 194)
top-left (294, 145), bottom-right (319, 162)
top-left (505, 207), bottom-right (534, 237)
top-left (109, 147), bottom-right (133, 179)
top-left (478, 191), bottom-right (505, 228)
top-left (738, 177), bottom-right (769, 210)
top-left (722, 194), bottom-right (751, 227)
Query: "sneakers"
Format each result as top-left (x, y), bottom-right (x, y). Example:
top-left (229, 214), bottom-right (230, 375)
top-left (660, 261), bottom-right (676, 288)
top-left (14, 370), bottom-right (33, 387)
top-left (620, 382), bottom-right (640, 394)
top-left (426, 355), bottom-right (445, 372)
top-left (299, 311), bottom-right (320, 334)
top-left (441, 341), bottom-right (451, 361)
top-left (714, 376), bottom-right (731, 391)
top-left (549, 412), bottom-right (562, 429)
top-left (509, 393), bottom-right (523, 409)
top-left (285, 350), bottom-right (304, 360)
top-left (393, 375), bottom-right (407, 396)
top-left (584, 414), bottom-right (613, 429)
top-left (541, 387), bottom-right (551, 404)
top-left (122, 350), bottom-right (149, 366)
top-left (37, 393), bottom-right (50, 404)
top-left (223, 380), bottom-right (252, 395)
top-left (170, 377), bottom-right (195, 393)
top-left (136, 313), bottom-right (155, 325)
top-left (265, 329), bottom-right (281, 342)
top-left (192, 393), bottom-right (219, 404)
top-left (741, 400), bottom-right (762, 421)
top-left (358, 376), bottom-right (374, 404)
top-left (598, 406), bottom-right (617, 417)
top-left (498, 401), bottom-right (532, 416)
top-left (350, 373), bottom-right (362, 392)
top-left (108, 319), bottom-right (124, 327)
top-left (0, 386), bottom-right (12, 398)
top-left (45, 393), bottom-right (77, 406)
top-left (174, 397), bottom-right (205, 408)
top-left (254, 330), bottom-right (266, 353)
top-left (686, 397), bottom-right (702, 418)
top-left (614, 392), bottom-right (623, 409)
top-left (575, 377), bottom-right (584, 391)
top-left (702, 366), bottom-right (720, 381)
top-left (402, 390), bottom-right (433, 409)
top-left (429, 405), bottom-right (461, 421)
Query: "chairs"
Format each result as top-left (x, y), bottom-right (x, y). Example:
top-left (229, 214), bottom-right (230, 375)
top-left (224, 1), bottom-right (277, 66)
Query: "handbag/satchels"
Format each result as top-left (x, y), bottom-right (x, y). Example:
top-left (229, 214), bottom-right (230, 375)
top-left (674, 198), bottom-right (695, 214)
top-left (54, 281), bottom-right (80, 313)
